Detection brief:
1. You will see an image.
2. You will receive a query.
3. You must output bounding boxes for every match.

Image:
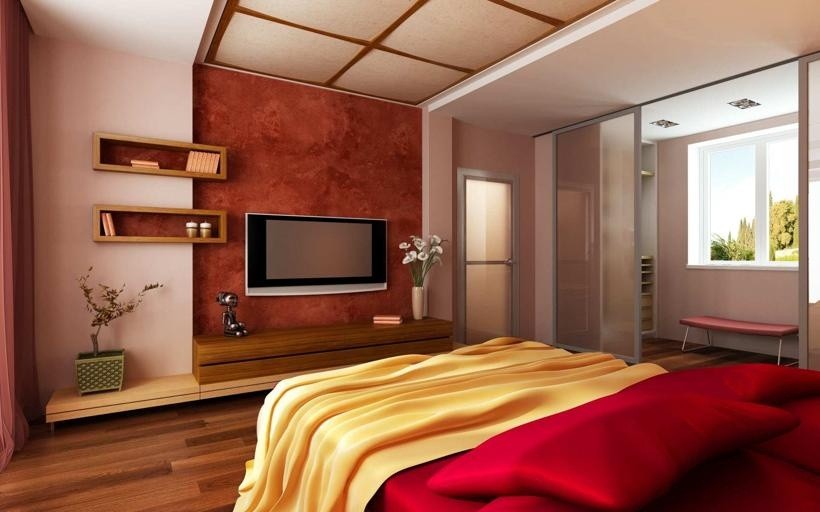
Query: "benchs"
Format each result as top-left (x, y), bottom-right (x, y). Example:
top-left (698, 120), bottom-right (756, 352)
top-left (678, 316), bottom-right (799, 367)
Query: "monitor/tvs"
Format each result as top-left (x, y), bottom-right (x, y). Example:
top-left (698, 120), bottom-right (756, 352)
top-left (244, 211), bottom-right (387, 297)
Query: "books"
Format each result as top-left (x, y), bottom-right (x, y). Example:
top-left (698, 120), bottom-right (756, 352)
top-left (101, 213), bottom-right (116, 236)
top-left (131, 159), bottom-right (159, 169)
top-left (186, 150), bottom-right (220, 174)
top-left (373, 315), bottom-right (403, 324)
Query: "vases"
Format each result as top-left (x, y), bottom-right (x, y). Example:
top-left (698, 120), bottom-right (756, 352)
top-left (412, 286), bottom-right (425, 321)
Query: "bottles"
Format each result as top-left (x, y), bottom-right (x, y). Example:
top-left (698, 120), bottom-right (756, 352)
top-left (186, 220), bottom-right (211, 238)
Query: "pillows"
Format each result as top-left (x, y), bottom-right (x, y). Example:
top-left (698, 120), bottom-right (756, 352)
top-left (424, 377), bottom-right (796, 510)
top-left (666, 363), bottom-right (820, 403)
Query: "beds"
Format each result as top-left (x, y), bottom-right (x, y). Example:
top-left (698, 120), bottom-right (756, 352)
top-left (269, 335), bottom-right (819, 512)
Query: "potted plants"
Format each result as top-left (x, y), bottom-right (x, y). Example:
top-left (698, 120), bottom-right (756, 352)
top-left (73, 266), bottom-right (167, 396)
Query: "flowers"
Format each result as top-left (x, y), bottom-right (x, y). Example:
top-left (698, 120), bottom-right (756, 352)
top-left (398, 233), bottom-right (448, 287)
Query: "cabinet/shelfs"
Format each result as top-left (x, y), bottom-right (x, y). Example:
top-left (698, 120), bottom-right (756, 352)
top-left (639, 140), bottom-right (658, 338)
top-left (91, 130), bottom-right (228, 183)
top-left (91, 202), bottom-right (228, 246)
